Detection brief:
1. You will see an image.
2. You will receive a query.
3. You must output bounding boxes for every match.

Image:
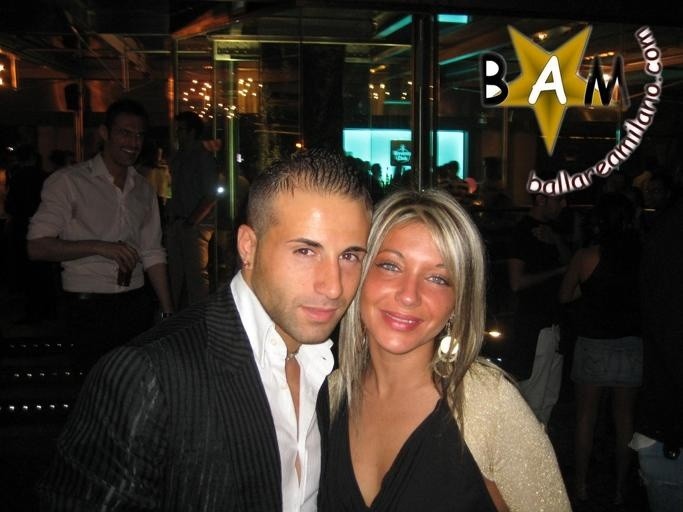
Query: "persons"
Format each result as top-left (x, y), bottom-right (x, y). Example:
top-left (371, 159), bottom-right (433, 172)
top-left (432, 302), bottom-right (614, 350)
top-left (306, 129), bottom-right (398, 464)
top-left (1, 83), bottom-right (683, 511)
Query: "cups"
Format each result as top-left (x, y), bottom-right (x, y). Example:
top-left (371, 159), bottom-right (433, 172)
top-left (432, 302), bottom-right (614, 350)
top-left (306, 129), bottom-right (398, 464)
top-left (116, 270), bottom-right (133, 287)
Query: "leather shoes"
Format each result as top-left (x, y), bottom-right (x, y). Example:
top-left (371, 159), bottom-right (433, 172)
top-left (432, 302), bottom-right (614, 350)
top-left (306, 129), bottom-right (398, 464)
top-left (663, 445), bottom-right (680, 459)
